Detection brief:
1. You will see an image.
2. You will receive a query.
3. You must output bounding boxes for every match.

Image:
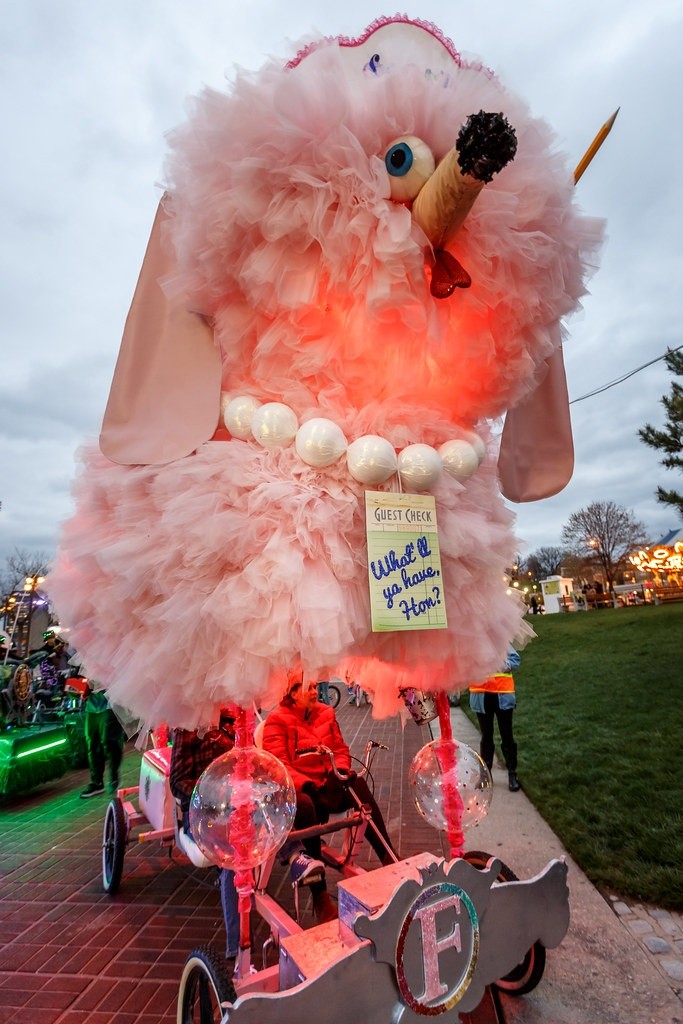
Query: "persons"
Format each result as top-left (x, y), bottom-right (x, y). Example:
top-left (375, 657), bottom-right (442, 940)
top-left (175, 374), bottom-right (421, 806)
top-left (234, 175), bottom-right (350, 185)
top-left (469, 641), bottom-right (522, 793)
top-left (170, 698), bottom-right (325, 984)
top-left (261, 667), bottom-right (403, 924)
top-left (531, 594), bottom-right (538, 614)
top-left (579, 581), bottom-right (605, 609)
top-left (78, 681), bottom-right (125, 798)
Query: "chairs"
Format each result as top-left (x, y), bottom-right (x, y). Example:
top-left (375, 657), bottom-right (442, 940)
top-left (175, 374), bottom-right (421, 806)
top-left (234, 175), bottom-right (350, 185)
top-left (174, 796), bottom-right (215, 867)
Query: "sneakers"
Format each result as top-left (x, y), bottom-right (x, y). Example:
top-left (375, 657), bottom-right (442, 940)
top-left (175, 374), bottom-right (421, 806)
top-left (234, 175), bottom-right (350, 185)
top-left (233, 963), bottom-right (259, 981)
top-left (289, 853), bottom-right (325, 888)
top-left (108, 773), bottom-right (119, 794)
top-left (80, 781), bottom-right (106, 798)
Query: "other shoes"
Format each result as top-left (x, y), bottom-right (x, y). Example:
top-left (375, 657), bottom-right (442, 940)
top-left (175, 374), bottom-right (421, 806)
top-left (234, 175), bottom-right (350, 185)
top-left (507, 770), bottom-right (522, 793)
top-left (476, 772), bottom-right (493, 790)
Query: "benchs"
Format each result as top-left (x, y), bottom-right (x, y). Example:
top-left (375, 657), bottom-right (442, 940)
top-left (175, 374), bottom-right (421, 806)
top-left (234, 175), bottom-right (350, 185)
top-left (561, 592), bottom-right (611, 609)
top-left (653, 586), bottom-right (683, 605)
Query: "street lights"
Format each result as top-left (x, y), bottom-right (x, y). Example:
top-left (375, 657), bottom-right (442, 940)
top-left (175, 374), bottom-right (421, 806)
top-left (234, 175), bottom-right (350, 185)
top-left (23, 574), bottom-right (45, 658)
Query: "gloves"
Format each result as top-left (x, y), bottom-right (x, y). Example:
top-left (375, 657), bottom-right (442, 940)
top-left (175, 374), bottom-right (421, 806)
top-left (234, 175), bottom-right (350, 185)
top-left (326, 766), bottom-right (345, 790)
top-left (303, 782), bottom-right (318, 799)
